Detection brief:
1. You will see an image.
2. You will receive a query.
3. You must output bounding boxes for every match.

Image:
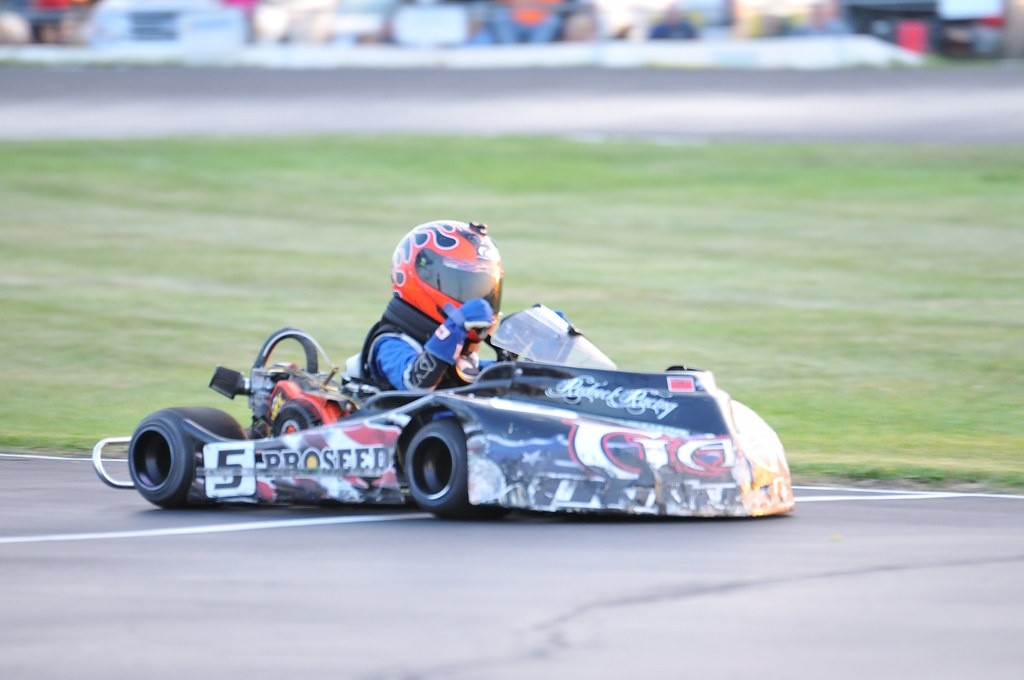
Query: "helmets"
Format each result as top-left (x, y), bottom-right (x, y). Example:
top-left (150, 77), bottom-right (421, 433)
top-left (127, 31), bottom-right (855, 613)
top-left (391, 219), bottom-right (505, 345)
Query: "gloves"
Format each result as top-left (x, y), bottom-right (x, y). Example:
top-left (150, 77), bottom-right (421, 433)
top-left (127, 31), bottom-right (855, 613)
top-left (423, 297), bottom-right (494, 367)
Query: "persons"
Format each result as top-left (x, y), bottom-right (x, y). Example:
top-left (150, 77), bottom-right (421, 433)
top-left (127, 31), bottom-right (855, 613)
top-left (563, 0), bottom-right (851, 39)
top-left (360, 219), bottom-right (502, 392)
